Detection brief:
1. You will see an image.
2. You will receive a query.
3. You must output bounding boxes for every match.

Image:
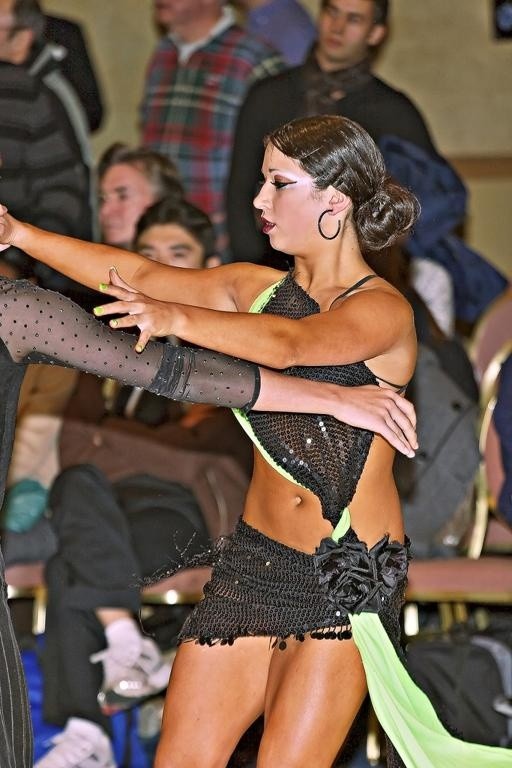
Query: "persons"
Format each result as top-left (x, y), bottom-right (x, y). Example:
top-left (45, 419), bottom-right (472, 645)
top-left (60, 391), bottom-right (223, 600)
top-left (0, 1), bottom-right (469, 768)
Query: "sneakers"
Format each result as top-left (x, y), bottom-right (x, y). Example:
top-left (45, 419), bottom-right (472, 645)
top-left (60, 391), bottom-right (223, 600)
top-left (32, 716), bottom-right (118, 768)
top-left (89, 636), bottom-right (171, 717)
